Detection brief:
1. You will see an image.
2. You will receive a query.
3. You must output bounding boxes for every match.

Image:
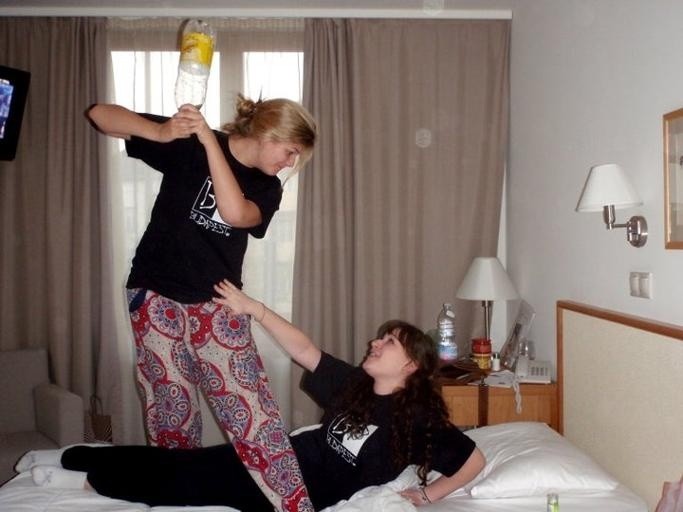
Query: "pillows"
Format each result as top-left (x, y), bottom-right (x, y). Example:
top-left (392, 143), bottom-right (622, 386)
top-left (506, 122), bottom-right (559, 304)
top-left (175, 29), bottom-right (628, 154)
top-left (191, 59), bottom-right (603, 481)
top-left (447, 421), bottom-right (617, 498)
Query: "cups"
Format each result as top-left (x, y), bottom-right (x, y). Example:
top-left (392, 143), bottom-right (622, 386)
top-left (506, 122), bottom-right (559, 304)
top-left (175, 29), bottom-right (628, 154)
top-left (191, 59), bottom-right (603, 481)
top-left (518, 341), bottom-right (537, 359)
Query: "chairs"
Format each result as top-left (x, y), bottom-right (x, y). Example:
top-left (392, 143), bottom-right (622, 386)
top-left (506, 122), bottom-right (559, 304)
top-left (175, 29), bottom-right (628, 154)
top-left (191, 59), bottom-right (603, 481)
top-left (1, 347), bottom-right (87, 485)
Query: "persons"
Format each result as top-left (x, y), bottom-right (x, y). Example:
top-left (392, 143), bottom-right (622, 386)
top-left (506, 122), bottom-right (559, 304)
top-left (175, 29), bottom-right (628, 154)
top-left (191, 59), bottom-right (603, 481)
top-left (15, 278), bottom-right (484, 511)
top-left (87, 89), bottom-right (318, 511)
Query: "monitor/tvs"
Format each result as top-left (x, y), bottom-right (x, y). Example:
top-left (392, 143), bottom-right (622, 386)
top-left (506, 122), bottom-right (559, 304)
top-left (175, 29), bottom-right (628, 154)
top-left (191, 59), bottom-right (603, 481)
top-left (0, 64), bottom-right (31, 161)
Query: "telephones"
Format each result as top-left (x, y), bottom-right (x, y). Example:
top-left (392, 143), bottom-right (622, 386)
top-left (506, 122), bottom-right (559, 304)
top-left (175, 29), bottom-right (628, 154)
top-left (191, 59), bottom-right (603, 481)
top-left (516, 359), bottom-right (553, 384)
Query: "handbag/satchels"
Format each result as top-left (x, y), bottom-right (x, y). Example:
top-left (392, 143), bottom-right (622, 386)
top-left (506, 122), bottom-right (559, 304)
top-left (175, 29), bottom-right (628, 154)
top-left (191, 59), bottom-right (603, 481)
top-left (433, 359), bottom-right (488, 386)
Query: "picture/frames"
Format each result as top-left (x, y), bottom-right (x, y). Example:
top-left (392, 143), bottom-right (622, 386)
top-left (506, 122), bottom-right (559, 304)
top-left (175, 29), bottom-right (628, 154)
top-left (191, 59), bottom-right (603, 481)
top-left (663, 109), bottom-right (683, 252)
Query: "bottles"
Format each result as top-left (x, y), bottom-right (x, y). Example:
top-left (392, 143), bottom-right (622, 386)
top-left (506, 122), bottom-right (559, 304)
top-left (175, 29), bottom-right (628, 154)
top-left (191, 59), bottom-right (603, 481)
top-left (436, 302), bottom-right (459, 359)
top-left (545, 492), bottom-right (560, 510)
top-left (491, 353), bottom-right (503, 370)
top-left (470, 339), bottom-right (492, 370)
top-left (174, 15), bottom-right (216, 113)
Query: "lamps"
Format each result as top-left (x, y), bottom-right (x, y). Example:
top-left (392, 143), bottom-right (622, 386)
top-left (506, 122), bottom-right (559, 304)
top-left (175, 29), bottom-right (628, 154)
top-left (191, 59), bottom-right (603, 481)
top-left (575, 162), bottom-right (649, 248)
top-left (455, 255), bottom-right (520, 341)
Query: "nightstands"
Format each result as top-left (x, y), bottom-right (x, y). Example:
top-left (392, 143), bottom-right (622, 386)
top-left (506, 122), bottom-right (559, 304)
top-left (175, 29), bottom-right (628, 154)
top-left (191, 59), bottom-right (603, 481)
top-left (430, 368), bottom-right (558, 430)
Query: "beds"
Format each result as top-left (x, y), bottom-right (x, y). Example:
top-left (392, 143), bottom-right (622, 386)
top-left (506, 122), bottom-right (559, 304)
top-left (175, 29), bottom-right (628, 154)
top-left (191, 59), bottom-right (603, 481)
top-left (0, 300), bottom-right (683, 512)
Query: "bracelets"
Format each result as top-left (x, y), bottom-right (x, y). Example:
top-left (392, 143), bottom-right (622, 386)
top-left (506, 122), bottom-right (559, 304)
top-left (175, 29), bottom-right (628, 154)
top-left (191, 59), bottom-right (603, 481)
top-left (419, 487), bottom-right (431, 504)
top-left (254, 302), bottom-right (264, 323)
